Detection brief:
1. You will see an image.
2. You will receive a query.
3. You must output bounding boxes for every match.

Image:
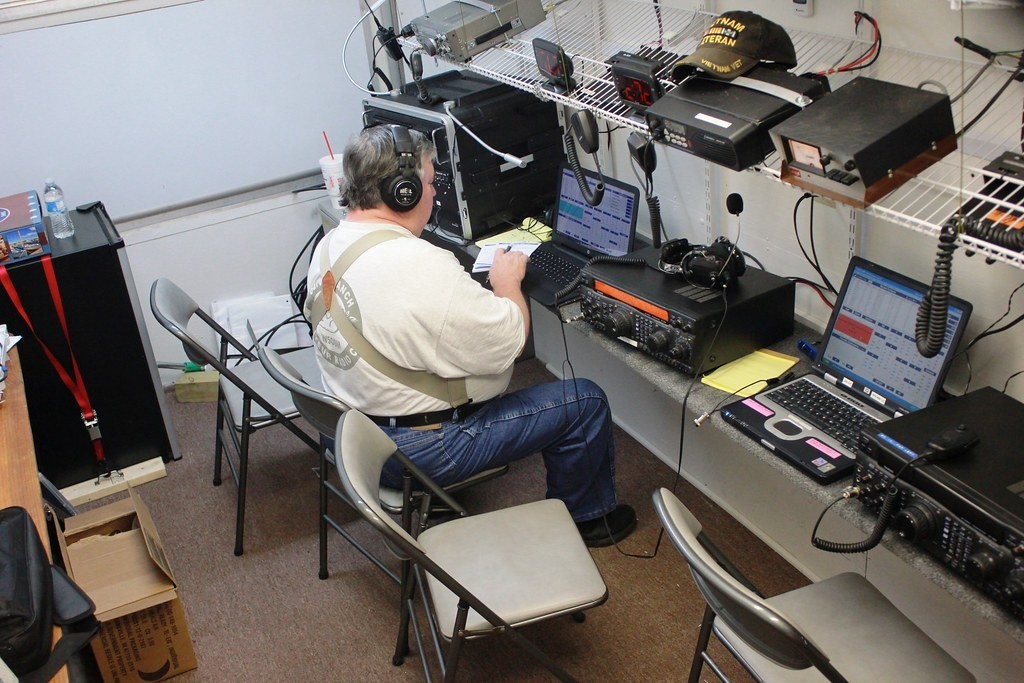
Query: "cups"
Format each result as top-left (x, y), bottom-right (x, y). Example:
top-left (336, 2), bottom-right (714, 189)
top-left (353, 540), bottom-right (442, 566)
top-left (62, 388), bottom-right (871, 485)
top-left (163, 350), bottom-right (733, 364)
top-left (318, 154), bottom-right (350, 209)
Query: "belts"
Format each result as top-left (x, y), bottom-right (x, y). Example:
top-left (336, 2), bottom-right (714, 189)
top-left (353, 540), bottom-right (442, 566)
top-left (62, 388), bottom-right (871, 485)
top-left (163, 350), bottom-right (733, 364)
top-left (365, 399), bottom-right (474, 427)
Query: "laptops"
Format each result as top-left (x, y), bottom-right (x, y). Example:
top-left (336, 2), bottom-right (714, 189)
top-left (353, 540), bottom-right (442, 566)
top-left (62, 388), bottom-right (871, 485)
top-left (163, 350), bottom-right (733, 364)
top-left (720, 256), bottom-right (973, 485)
top-left (519, 162), bottom-right (640, 307)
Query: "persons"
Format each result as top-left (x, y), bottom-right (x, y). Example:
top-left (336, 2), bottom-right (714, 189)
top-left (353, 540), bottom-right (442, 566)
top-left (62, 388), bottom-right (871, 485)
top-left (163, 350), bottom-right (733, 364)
top-left (303, 123), bottom-right (636, 546)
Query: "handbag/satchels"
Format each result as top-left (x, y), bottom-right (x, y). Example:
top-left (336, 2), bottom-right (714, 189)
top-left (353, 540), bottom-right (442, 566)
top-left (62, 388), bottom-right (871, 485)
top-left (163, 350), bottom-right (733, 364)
top-left (0, 507), bottom-right (102, 683)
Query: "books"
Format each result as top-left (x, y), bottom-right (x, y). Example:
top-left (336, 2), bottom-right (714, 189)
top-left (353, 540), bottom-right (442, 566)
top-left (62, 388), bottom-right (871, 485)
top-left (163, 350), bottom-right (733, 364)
top-left (475, 217), bottom-right (554, 249)
top-left (701, 348), bottom-right (801, 397)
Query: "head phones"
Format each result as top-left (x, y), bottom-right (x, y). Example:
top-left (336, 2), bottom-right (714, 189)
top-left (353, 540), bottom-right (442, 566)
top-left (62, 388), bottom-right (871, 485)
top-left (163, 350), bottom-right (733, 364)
top-left (657, 238), bottom-right (747, 288)
top-left (380, 125), bottom-right (423, 212)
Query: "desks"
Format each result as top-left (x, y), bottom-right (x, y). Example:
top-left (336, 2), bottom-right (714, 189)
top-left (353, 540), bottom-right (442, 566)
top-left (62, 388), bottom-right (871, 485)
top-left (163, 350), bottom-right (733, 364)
top-left (307, 195), bottom-right (1024, 683)
top-left (0, 332), bottom-right (74, 683)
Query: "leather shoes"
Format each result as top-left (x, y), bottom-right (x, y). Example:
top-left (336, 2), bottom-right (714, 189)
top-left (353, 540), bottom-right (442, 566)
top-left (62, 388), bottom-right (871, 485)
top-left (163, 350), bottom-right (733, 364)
top-left (576, 505), bottom-right (636, 547)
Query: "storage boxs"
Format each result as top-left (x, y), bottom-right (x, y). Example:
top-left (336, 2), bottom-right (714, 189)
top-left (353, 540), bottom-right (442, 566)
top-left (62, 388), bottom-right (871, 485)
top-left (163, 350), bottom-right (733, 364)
top-left (0, 190), bottom-right (52, 267)
top-left (59, 481), bottom-right (199, 683)
top-left (219, 345), bottom-right (325, 432)
top-left (175, 370), bottom-right (219, 404)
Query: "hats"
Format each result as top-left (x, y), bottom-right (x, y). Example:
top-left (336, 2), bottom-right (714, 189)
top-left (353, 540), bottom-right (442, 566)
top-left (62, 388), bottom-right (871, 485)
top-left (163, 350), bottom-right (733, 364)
top-left (670, 11), bottom-right (797, 80)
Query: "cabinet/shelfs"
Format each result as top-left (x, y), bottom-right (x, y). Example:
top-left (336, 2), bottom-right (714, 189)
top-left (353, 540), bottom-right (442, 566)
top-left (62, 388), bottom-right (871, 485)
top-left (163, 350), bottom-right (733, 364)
top-left (0, 200), bottom-right (184, 509)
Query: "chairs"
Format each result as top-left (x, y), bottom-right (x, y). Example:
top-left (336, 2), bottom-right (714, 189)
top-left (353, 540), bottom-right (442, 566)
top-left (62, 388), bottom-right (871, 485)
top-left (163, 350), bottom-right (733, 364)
top-left (149, 274), bottom-right (508, 623)
top-left (335, 407), bottom-right (609, 683)
top-left (651, 487), bottom-right (977, 683)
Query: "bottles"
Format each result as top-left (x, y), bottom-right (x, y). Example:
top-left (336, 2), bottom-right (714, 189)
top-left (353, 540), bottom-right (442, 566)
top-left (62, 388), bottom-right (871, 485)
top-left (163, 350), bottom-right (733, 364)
top-left (43, 178), bottom-right (75, 239)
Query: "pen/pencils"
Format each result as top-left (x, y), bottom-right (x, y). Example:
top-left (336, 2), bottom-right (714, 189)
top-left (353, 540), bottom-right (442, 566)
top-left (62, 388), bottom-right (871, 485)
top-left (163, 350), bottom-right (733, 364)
top-left (485, 242), bottom-right (514, 284)
